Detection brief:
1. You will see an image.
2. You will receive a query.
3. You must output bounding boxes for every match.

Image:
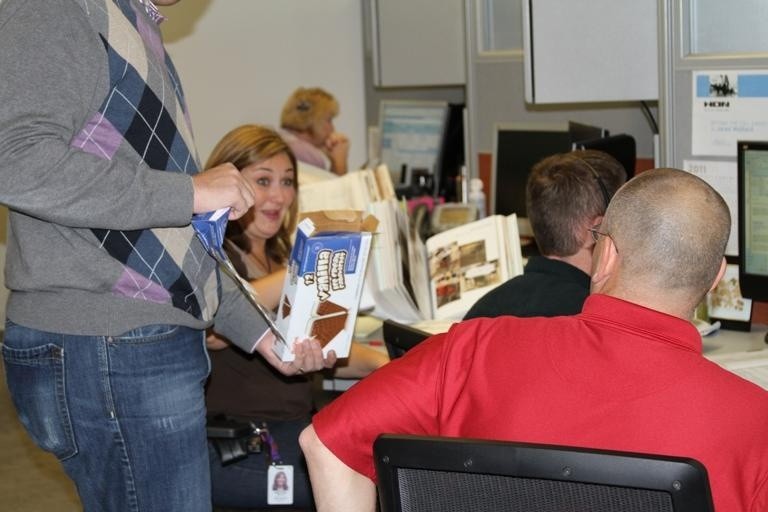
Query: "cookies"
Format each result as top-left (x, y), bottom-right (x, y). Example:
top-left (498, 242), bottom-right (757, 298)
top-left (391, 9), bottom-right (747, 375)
top-left (281, 295), bottom-right (292, 318)
top-left (310, 300), bottom-right (349, 349)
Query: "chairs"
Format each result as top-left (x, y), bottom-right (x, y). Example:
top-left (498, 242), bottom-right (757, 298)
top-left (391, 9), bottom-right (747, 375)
top-left (373, 433), bottom-right (714, 511)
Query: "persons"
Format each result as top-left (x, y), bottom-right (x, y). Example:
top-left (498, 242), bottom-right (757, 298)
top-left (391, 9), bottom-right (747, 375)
top-left (301, 169), bottom-right (767, 512)
top-left (0, 0), bottom-right (255, 512)
top-left (274, 86), bottom-right (350, 176)
top-left (201, 124), bottom-right (390, 512)
top-left (462, 149), bottom-right (629, 321)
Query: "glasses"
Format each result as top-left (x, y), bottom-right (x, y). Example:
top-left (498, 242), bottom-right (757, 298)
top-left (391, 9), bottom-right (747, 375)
top-left (586, 224), bottom-right (619, 255)
